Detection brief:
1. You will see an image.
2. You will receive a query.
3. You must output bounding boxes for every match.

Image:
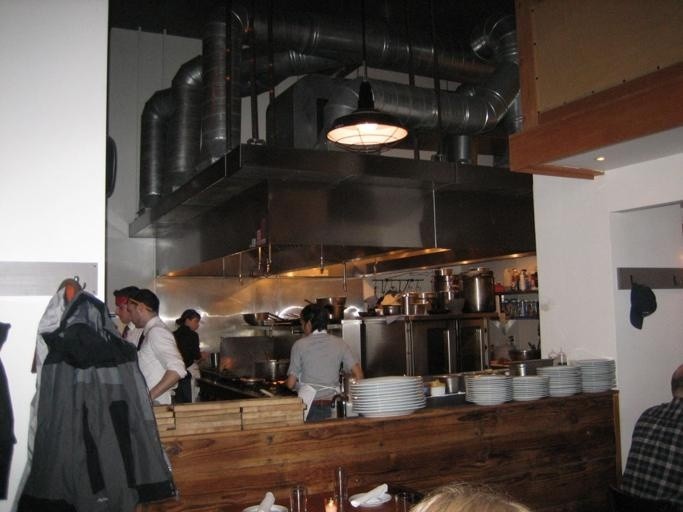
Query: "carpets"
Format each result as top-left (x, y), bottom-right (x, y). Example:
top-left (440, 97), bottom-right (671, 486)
top-left (122, 325), bottom-right (131, 339)
top-left (136, 329), bottom-right (146, 351)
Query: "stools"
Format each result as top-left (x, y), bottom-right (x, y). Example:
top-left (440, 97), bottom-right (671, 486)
top-left (311, 400), bottom-right (332, 406)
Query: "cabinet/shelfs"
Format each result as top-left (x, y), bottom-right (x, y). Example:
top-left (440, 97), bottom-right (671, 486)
top-left (498, 267), bottom-right (539, 317)
top-left (333, 467), bottom-right (349, 502)
top-left (557, 347), bottom-right (567, 365)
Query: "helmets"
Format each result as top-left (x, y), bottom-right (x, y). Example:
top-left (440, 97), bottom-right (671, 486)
top-left (349, 492), bottom-right (392, 509)
top-left (241, 503), bottom-right (288, 512)
top-left (465, 357), bottom-right (617, 407)
top-left (347, 375), bottom-right (427, 418)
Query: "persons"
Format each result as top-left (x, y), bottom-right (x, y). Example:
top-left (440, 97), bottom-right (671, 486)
top-left (113, 284), bottom-right (144, 350)
top-left (126, 289), bottom-right (188, 408)
top-left (618, 364), bottom-right (681, 510)
top-left (284, 303), bottom-right (365, 421)
top-left (173, 309), bottom-right (209, 405)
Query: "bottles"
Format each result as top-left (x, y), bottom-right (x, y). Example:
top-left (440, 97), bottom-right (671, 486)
top-left (629, 282), bottom-right (657, 329)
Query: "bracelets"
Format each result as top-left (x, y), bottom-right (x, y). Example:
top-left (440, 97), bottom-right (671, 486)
top-left (382, 291), bottom-right (466, 316)
top-left (241, 312), bottom-right (269, 327)
top-left (433, 268), bottom-right (454, 277)
top-left (316, 297), bottom-right (347, 306)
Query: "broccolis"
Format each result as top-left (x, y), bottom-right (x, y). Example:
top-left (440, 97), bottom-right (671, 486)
top-left (360, 310), bottom-right (491, 380)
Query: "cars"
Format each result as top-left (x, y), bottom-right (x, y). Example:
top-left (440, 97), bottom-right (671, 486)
top-left (289, 485), bottom-right (308, 512)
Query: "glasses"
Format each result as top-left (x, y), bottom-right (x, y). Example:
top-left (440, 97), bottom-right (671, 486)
top-left (326, 1), bottom-right (409, 149)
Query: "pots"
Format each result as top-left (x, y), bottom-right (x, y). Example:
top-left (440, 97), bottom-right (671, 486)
top-left (304, 298), bottom-right (347, 324)
top-left (430, 274), bottom-right (465, 291)
top-left (458, 269), bottom-right (497, 313)
top-left (253, 358), bottom-right (290, 383)
